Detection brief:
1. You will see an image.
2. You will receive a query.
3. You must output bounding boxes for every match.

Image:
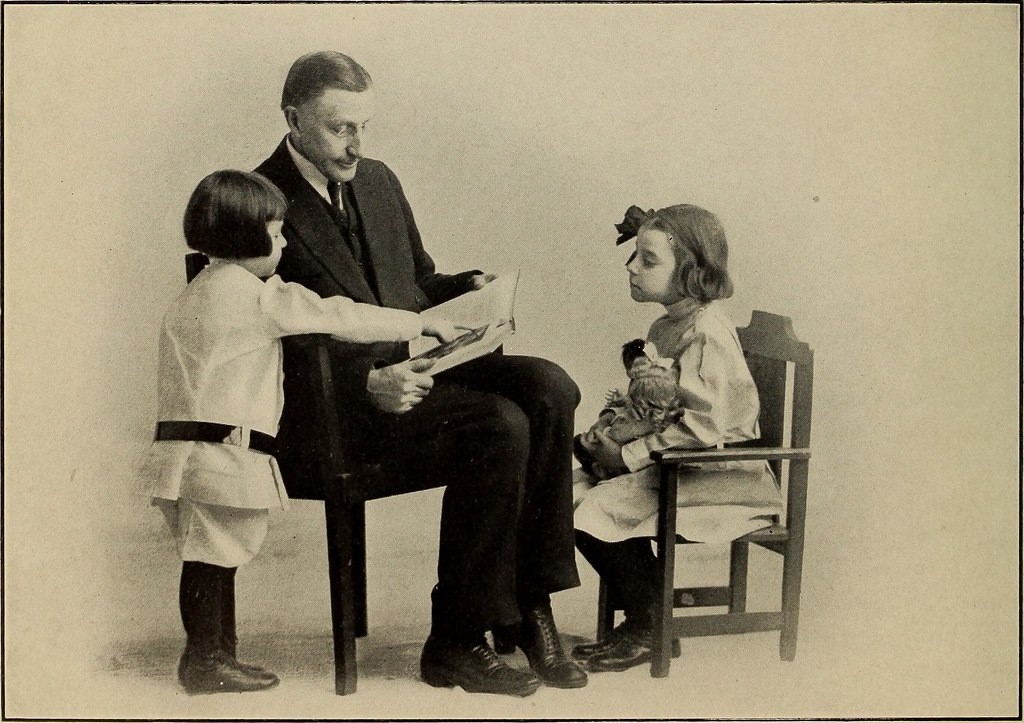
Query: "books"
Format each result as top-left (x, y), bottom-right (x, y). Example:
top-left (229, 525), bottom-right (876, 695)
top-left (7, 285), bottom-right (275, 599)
top-left (401, 268), bottom-right (520, 378)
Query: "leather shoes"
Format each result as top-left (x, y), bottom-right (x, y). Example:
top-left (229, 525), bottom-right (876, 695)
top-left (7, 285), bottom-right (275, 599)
top-left (494, 603), bottom-right (589, 689)
top-left (569, 621), bottom-right (637, 660)
top-left (587, 626), bottom-right (680, 672)
top-left (420, 639), bottom-right (541, 698)
top-left (177, 650), bottom-right (280, 696)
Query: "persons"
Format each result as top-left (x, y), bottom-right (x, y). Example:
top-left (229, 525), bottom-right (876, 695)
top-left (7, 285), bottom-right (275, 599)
top-left (571, 205), bottom-right (784, 672)
top-left (131, 171), bottom-right (475, 693)
top-left (254, 51), bottom-right (588, 697)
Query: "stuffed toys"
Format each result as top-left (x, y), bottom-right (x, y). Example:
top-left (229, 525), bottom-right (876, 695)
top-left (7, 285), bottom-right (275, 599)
top-left (572, 339), bottom-right (680, 480)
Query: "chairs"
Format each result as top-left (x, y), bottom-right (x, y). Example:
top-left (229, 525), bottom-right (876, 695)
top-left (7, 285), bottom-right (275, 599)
top-left (184, 251), bottom-right (514, 694)
top-left (596, 310), bottom-right (815, 677)
